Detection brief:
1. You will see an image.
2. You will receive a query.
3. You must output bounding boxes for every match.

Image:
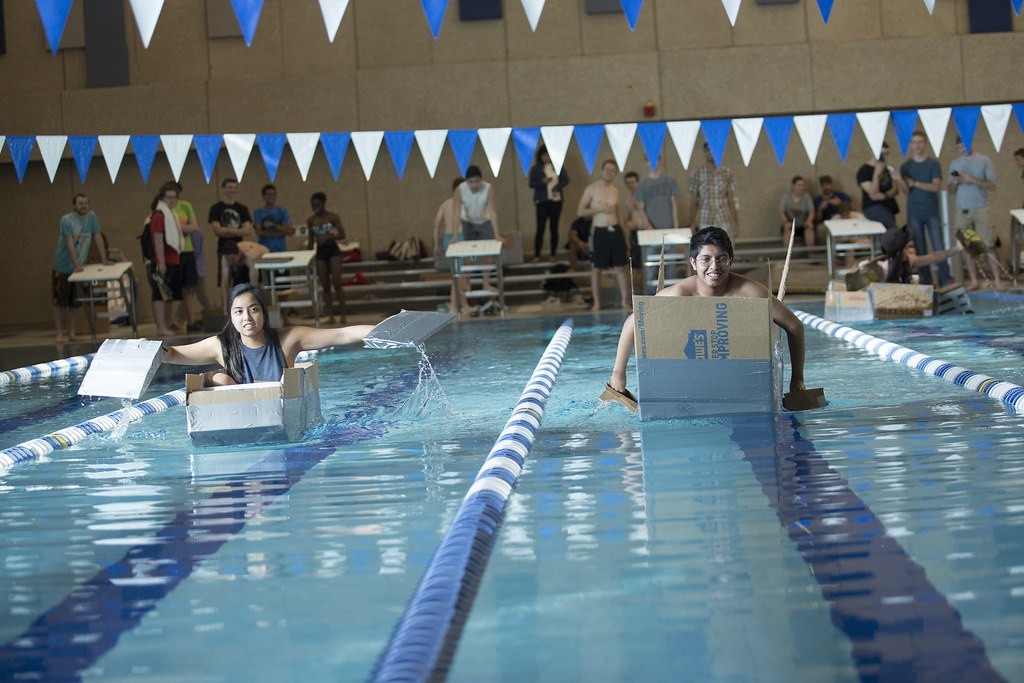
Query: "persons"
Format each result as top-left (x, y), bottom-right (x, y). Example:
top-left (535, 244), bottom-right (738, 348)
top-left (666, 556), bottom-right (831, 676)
top-left (209, 176), bottom-right (252, 317)
top-left (569, 205), bottom-right (592, 272)
top-left (1015, 148), bottom-right (1024, 178)
top-left (50, 192), bottom-right (115, 343)
top-left (635, 151), bottom-right (681, 295)
top-left (432, 175), bottom-right (478, 317)
top-left (778, 176), bottom-right (818, 263)
top-left (813, 176), bottom-right (852, 252)
top-left (623, 170), bottom-right (645, 270)
top-left (452, 165), bottom-right (508, 291)
top-left (145, 181), bottom-right (211, 337)
top-left (254, 184), bottom-right (295, 315)
top-left (576, 159), bottom-right (633, 311)
top-left (306, 191), bottom-right (347, 323)
top-left (948, 134), bottom-right (1006, 292)
top-left (891, 131), bottom-right (952, 288)
top-left (686, 143), bottom-right (740, 240)
top-left (607, 226), bottom-right (805, 395)
top-left (853, 225), bottom-right (966, 285)
top-left (529, 144), bottom-right (569, 261)
top-left (856, 142), bottom-right (900, 232)
top-left (159, 282), bottom-right (375, 385)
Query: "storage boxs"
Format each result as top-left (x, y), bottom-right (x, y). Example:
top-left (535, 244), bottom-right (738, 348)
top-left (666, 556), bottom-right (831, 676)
top-left (184, 359), bottom-right (321, 446)
top-left (629, 257), bottom-right (784, 422)
top-left (823, 279), bottom-right (935, 323)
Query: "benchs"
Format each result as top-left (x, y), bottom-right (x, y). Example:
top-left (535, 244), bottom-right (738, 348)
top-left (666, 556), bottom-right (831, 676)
top-left (262, 237), bottom-right (879, 314)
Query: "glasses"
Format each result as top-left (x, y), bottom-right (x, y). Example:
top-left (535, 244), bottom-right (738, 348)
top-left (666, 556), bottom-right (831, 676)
top-left (694, 257), bottom-right (733, 267)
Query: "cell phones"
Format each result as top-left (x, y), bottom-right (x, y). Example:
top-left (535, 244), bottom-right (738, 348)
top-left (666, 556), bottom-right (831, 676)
top-left (951, 171), bottom-right (958, 176)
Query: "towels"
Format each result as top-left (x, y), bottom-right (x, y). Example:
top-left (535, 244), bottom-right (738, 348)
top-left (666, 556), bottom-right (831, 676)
top-left (190, 229), bottom-right (209, 278)
top-left (153, 199), bottom-right (186, 258)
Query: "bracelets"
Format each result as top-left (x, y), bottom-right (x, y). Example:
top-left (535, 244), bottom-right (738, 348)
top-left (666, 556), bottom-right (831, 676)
top-left (912, 179), bottom-right (916, 188)
top-left (884, 193), bottom-right (888, 199)
